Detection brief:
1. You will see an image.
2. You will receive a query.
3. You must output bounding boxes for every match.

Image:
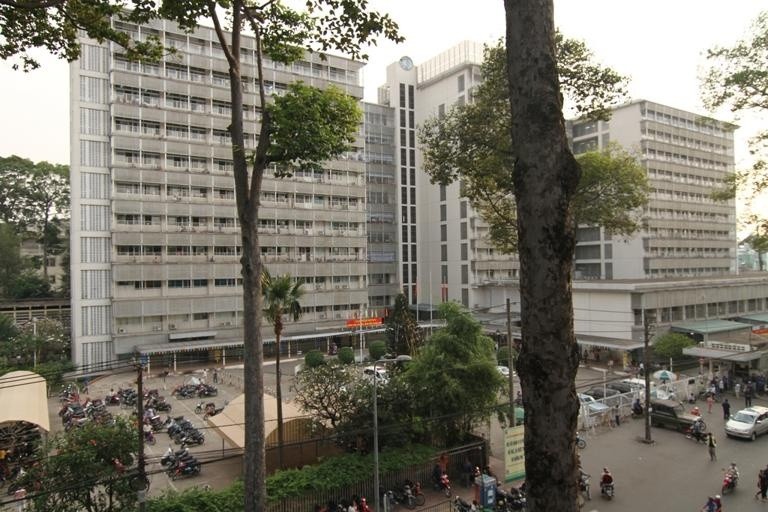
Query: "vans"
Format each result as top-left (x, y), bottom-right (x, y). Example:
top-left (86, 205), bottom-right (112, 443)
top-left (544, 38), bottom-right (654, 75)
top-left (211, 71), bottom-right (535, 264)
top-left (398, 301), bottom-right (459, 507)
top-left (725, 403), bottom-right (767, 443)
top-left (362, 365), bottom-right (388, 389)
top-left (294, 360), bottom-right (347, 399)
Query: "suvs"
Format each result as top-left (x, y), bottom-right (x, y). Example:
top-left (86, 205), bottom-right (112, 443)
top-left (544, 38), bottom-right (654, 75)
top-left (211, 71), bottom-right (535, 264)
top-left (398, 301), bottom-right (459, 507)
top-left (650, 401), bottom-right (700, 436)
top-left (576, 374), bottom-right (675, 416)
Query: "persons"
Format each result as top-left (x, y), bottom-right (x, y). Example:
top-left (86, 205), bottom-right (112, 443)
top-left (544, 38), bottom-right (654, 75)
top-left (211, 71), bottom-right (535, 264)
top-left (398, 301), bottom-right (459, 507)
top-left (107, 388), bottom-right (117, 405)
top-left (460, 456), bottom-right (473, 490)
top-left (471, 499), bottom-right (478, 512)
top-left (82, 379), bottom-right (89, 395)
top-left (202, 368), bottom-right (207, 384)
top-left (212, 369), bottom-right (218, 384)
top-left (575, 347), bottom-right (768, 512)
top-left (142, 421), bottom-right (153, 440)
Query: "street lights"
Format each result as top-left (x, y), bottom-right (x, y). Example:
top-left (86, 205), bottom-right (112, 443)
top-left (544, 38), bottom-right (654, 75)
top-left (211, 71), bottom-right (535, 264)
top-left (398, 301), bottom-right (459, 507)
top-left (127, 354), bottom-right (149, 479)
top-left (31, 317), bottom-right (38, 370)
top-left (372, 355), bottom-right (414, 509)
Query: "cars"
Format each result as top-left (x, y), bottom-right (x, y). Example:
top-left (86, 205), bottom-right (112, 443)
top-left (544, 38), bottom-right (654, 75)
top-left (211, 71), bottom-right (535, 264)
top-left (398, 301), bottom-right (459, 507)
top-left (495, 366), bottom-right (518, 379)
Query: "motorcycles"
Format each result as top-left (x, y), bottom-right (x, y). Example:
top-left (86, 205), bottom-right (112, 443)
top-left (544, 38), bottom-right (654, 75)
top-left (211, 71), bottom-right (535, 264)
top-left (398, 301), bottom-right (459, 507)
top-left (57, 374), bottom-right (225, 492)
top-left (0, 423), bottom-right (45, 497)
top-left (718, 467), bottom-right (738, 496)
top-left (600, 475), bottom-right (616, 499)
top-left (575, 425), bottom-right (592, 508)
top-left (385, 459), bottom-right (527, 512)
top-left (699, 501), bottom-right (723, 512)
top-left (687, 424), bottom-right (710, 443)
